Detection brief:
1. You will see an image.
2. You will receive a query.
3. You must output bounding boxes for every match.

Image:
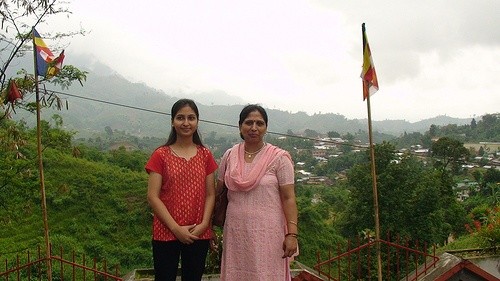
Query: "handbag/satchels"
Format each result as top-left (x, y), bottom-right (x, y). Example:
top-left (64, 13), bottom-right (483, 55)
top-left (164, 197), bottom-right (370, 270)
top-left (212, 149), bottom-right (232, 227)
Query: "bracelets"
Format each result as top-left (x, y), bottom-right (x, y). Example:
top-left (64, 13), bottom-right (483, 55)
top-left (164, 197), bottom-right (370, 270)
top-left (285, 233), bottom-right (298, 238)
top-left (288, 220), bottom-right (297, 225)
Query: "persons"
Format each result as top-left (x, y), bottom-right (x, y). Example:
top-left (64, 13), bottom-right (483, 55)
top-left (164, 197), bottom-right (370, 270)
top-left (215, 105), bottom-right (299, 281)
top-left (145, 98), bottom-right (217, 281)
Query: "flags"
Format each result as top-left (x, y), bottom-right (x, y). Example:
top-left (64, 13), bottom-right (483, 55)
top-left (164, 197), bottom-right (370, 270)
top-left (360, 28), bottom-right (379, 101)
top-left (34, 29), bottom-right (61, 78)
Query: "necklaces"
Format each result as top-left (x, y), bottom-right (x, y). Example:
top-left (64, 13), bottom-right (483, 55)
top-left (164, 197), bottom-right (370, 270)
top-left (244, 143), bottom-right (265, 158)
